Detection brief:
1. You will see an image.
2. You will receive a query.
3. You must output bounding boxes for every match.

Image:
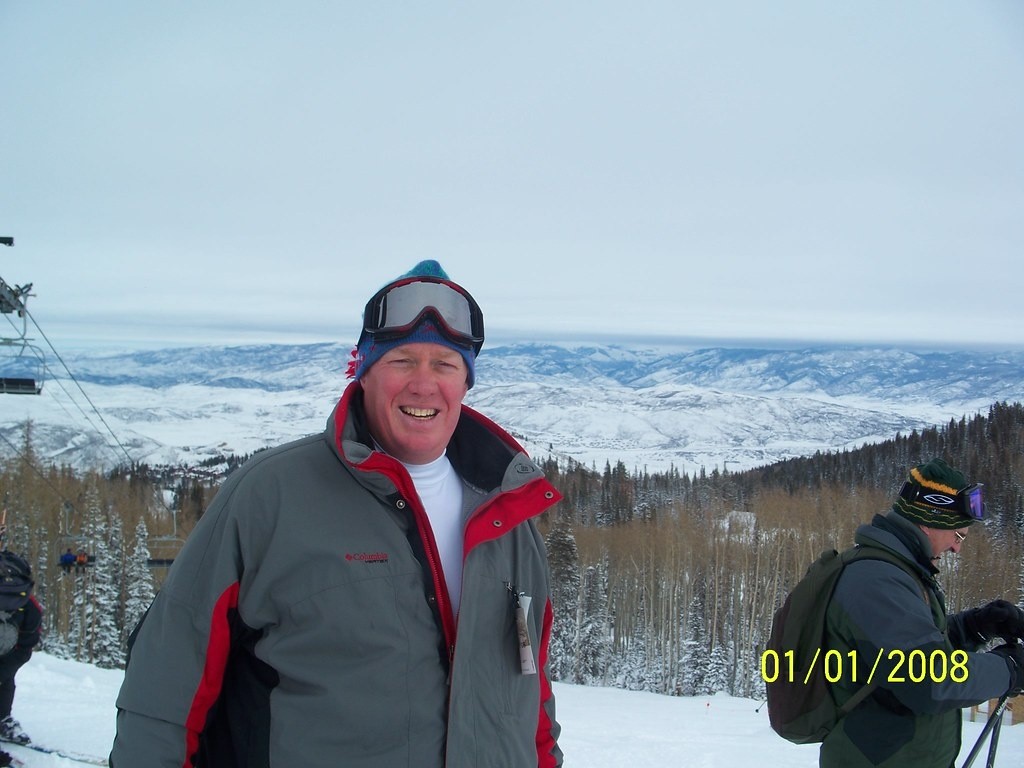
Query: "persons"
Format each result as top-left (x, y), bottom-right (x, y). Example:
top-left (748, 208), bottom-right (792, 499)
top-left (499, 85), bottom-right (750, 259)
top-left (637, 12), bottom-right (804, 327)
top-left (0, 551), bottom-right (44, 767)
top-left (60, 548), bottom-right (90, 576)
top-left (107, 260), bottom-right (564, 768)
top-left (819, 457), bottom-right (1024, 768)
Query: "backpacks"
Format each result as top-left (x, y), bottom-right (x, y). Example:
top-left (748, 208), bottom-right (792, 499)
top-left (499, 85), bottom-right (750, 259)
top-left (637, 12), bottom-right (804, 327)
top-left (0, 551), bottom-right (35, 610)
top-left (765, 547), bottom-right (935, 745)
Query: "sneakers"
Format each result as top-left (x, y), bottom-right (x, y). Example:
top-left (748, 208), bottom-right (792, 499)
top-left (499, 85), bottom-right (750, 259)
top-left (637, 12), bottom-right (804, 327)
top-left (0, 717), bottom-right (31, 745)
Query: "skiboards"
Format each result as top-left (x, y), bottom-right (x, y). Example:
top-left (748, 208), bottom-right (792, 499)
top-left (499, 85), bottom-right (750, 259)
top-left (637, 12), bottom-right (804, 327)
top-left (1, 739), bottom-right (110, 768)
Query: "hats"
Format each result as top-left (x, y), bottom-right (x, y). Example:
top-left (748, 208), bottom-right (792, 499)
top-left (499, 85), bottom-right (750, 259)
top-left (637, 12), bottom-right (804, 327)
top-left (356, 261), bottom-right (476, 391)
top-left (892, 457), bottom-right (976, 529)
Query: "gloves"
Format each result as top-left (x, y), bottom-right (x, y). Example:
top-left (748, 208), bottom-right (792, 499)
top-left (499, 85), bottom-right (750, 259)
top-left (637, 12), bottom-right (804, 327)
top-left (964, 600), bottom-right (1024, 646)
top-left (986, 642), bottom-right (1024, 697)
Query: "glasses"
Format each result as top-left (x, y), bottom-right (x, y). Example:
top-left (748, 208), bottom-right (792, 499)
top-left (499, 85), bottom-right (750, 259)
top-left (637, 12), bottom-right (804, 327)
top-left (953, 531), bottom-right (966, 543)
top-left (957, 483), bottom-right (985, 521)
top-left (362, 277), bottom-right (485, 348)
